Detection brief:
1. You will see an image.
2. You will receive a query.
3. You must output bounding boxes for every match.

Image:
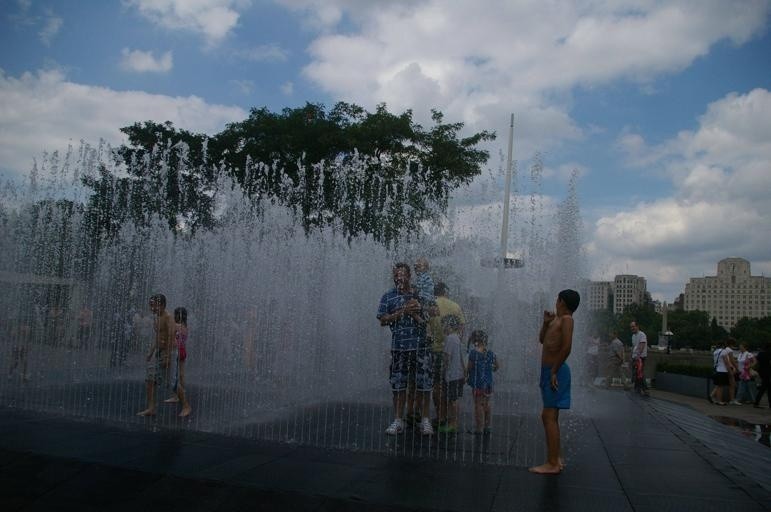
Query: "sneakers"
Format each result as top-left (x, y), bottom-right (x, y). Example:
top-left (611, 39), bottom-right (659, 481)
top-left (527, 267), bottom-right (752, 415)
top-left (419, 422), bottom-right (434, 436)
top-left (416, 413), bottom-right (422, 423)
top-left (384, 419), bottom-right (404, 435)
top-left (716, 401), bottom-right (727, 406)
top-left (438, 425), bottom-right (457, 433)
top-left (708, 394), bottom-right (715, 403)
top-left (729, 401), bottom-right (742, 406)
top-left (405, 414), bottom-right (417, 427)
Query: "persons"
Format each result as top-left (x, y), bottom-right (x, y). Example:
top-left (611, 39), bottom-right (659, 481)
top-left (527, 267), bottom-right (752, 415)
top-left (468, 329), bottom-right (502, 437)
top-left (377, 255), bottom-right (468, 438)
top-left (582, 318), bottom-right (771, 410)
top-left (527, 289), bottom-right (582, 476)
top-left (1, 305), bottom-right (280, 384)
top-left (162, 306), bottom-right (191, 404)
top-left (135, 293), bottom-right (194, 419)
